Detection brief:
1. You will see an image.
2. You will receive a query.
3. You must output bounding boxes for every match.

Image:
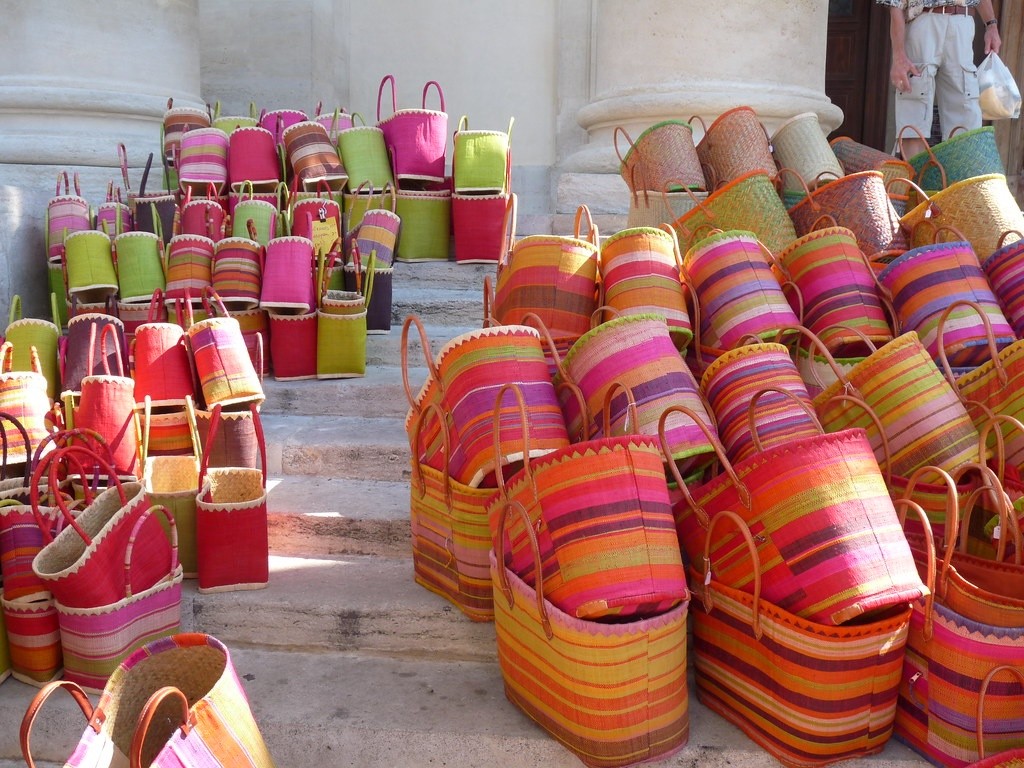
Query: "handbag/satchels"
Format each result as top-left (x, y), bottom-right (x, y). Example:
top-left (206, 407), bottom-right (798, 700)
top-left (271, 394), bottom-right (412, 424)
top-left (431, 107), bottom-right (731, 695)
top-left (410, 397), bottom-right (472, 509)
top-left (976, 49), bottom-right (1022, 120)
top-left (19, 633), bottom-right (272, 768)
top-left (0, 74), bottom-right (538, 694)
top-left (400, 105), bottom-right (1024, 768)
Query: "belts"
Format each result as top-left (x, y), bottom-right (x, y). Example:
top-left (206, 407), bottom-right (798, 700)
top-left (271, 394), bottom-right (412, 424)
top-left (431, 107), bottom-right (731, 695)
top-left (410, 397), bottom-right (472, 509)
top-left (922, 5), bottom-right (975, 18)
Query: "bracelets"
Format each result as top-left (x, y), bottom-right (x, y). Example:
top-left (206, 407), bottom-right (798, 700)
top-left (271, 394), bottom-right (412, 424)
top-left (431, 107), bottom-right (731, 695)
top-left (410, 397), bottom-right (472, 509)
top-left (984, 18), bottom-right (997, 28)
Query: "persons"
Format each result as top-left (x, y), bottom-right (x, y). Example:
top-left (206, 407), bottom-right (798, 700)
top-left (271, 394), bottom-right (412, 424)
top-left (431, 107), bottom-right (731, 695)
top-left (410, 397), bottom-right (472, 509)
top-left (876, 0), bottom-right (1003, 164)
